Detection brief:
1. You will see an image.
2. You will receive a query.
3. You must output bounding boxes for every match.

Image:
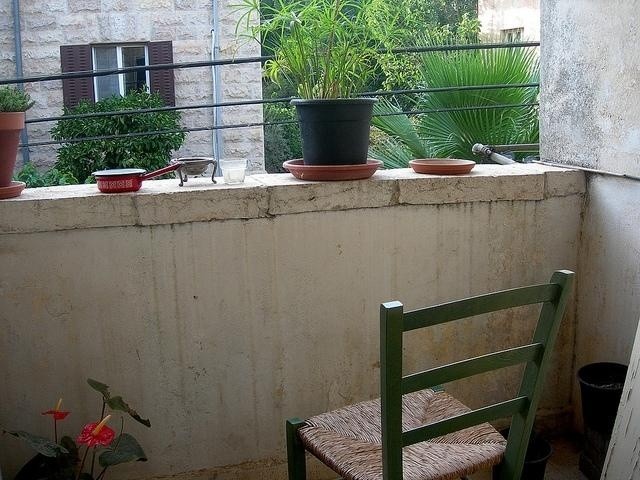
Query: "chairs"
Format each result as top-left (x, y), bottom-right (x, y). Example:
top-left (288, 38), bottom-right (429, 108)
top-left (286, 270), bottom-right (574, 480)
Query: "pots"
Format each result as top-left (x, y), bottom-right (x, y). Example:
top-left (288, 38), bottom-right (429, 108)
top-left (91, 163), bottom-right (182, 193)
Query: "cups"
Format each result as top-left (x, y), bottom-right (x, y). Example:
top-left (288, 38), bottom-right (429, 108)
top-left (220, 157), bottom-right (248, 184)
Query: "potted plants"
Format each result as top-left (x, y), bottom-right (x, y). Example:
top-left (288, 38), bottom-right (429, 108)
top-left (0, 85), bottom-right (35, 200)
top-left (227, 0), bottom-right (420, 181)
